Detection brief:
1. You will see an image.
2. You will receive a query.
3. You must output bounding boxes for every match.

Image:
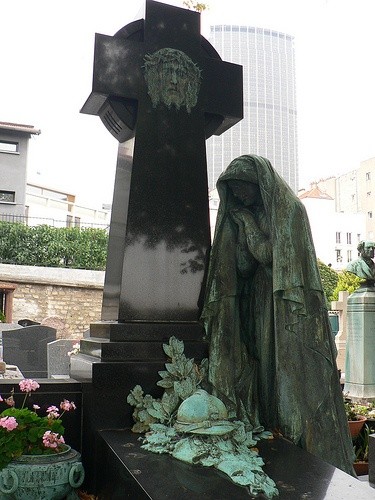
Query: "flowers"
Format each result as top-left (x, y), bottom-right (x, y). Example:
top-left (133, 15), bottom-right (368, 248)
top-left (0, 379), bottom-right (76, 471)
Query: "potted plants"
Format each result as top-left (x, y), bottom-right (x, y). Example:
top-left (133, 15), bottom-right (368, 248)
top-left (353, 424), bottom-right (374, 476)
top-left (344, 403), bottom-right (367, 435)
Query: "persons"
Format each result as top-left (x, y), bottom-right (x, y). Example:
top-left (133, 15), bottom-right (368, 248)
top-left (208, 153), bottom-right (349, 465)
top-left (145, 48), bottom-right (200, 109)
top-left (348, 239), bottom-right (375, 286)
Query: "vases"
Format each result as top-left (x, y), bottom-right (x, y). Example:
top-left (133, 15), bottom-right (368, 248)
top-left (0, 443), bottom-right (85, 500)
